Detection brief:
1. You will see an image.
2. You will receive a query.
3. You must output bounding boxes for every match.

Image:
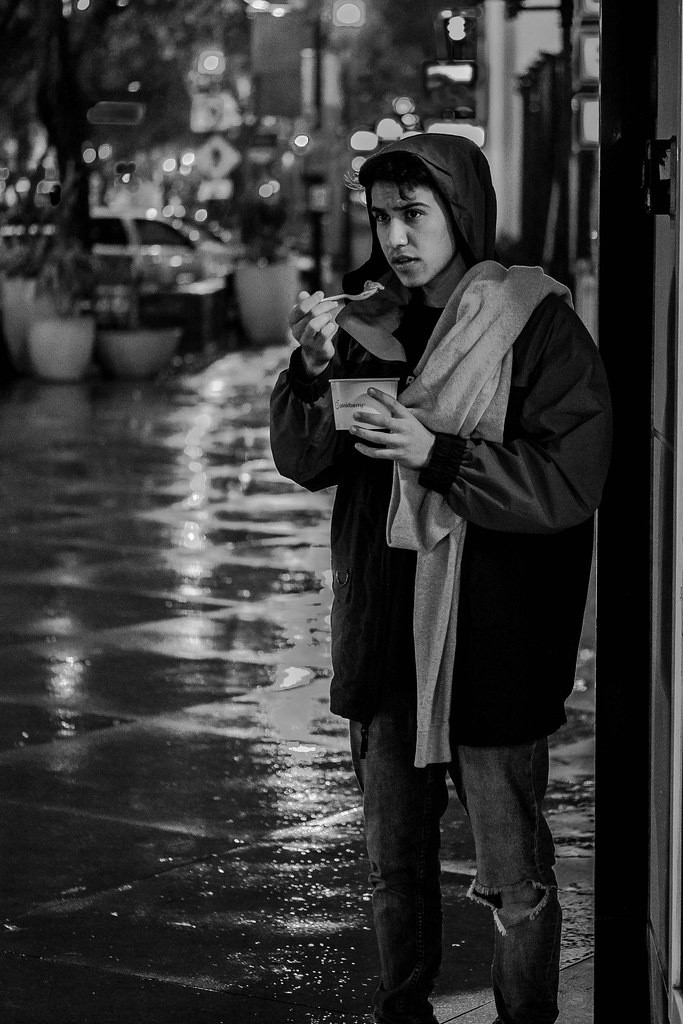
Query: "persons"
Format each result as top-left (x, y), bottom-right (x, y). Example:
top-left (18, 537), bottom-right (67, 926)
top-left (270, 133), bottom-right (613, 1024)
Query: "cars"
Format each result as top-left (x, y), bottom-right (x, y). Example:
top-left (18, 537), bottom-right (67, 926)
top-left (3, 212), bottom-right (245, 288)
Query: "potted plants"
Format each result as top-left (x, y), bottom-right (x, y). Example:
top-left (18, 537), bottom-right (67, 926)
top-left (235, 226), bottom-right (300, 346)
top-left (96, 266), bottom-right (183, 380)
top-left (0, 158), bottom-right (102, 354)
top-left (25, 248), bottom-right (105, 380)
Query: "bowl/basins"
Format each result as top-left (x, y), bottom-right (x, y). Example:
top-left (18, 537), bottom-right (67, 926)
top-left (329, 378), bottom-right (400, 430)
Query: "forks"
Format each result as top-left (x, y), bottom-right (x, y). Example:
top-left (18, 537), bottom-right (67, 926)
top-left (292, 288), bottom-right (378, 308)
